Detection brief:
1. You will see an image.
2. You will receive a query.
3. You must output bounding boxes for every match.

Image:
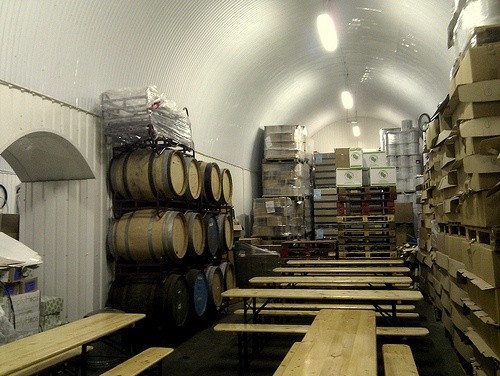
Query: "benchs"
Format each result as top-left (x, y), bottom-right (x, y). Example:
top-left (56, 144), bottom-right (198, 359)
top-left (99, 347), bottom-right (175, 376)
top-left (213, 322), bottom-right (429, 376)
top-left (6, 346), bottom-right (94, 376)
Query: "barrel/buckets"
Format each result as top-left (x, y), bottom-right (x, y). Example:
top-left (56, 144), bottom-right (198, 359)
top-left (109, 262), bottom-right (237, 327)
top-left (109, 148), bottom-right (188, 202)
top-left (202, 213), bottom-right (219, 256)
top-left (182, 212), bottom-right (206, 258)
top-left (108, 208), bottom-right (189, 263)
top-left (215, 214), bottom-right (234, 252)
top-left (179, 156), bottom-right (202, 201)
top-left (198, 161), bottom-right (222, 203)
top-left (218, 168), bottom-right (233, 205)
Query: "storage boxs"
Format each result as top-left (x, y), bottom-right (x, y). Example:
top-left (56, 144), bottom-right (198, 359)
top-left (0, 268), bottom-right (65, 333)
top-left (252, 125), bottom-right (312, 236)
top-left (414, 34), bottom-right (500, 376)
top-left (335, 148), bottom-right (397, 188)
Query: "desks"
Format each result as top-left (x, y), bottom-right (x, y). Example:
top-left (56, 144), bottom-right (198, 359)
top-left (0, 313), bottom-right (146, 376)
top-left (221, 287), bottom-right (423, 376)
top-left (279, 308), bottom-right (376, 376)
top-left (248, 276), bottom-right (412, 289)
top-left (286, 259), bottom-right (404, 267)
top-left (271, 267), bottom-right (410, 276)
